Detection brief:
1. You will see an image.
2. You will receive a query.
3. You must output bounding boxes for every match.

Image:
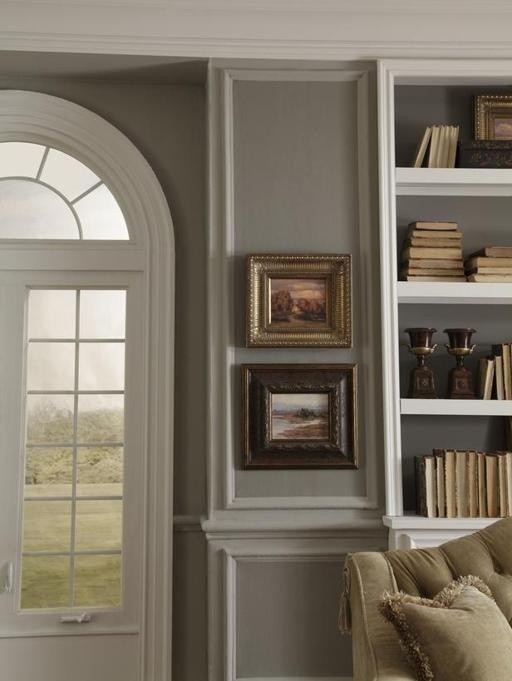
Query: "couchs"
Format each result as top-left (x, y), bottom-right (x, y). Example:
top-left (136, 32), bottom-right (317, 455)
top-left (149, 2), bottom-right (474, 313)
top-left (337, 515), bottom-right (512, 681)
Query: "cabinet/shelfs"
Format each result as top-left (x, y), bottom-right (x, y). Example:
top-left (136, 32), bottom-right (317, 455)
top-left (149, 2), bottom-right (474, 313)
top-left (376, 57), bottom-right (511, 549)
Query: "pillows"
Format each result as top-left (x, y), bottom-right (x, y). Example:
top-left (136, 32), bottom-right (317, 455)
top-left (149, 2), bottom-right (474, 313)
top-left (377, 574), bottom-right (512, 681)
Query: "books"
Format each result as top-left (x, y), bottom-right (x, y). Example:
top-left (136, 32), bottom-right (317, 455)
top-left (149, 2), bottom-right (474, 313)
top-left (475, 343), bottom-right (512, 401)
top-left (402, 221), bottom-right (512, 283)
top-left (412, 448), bottom-right (512, 519)
top-left (411, 124), bottom-right (460, 168)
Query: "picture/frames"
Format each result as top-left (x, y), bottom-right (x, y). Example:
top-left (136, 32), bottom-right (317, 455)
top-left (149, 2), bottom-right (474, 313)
top-left (473, 94), bottom-right (512, 140)
top-left (242, 255), bottom-right (357, 470)
